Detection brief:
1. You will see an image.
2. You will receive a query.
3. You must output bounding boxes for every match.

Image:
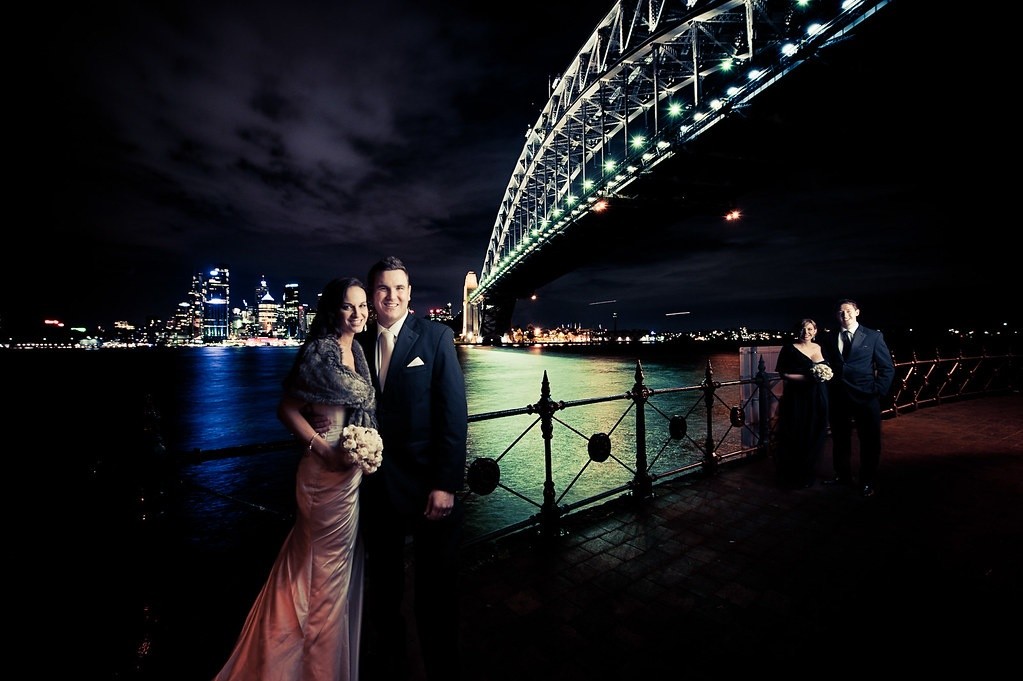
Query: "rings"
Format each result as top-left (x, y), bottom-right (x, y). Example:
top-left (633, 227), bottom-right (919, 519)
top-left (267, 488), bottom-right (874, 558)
top-left (442, 513), bottom-right (446, 517)
top-left (770, 315), bottom-right (834, 496)
top-left (208, 253), bottom-right (470, 680)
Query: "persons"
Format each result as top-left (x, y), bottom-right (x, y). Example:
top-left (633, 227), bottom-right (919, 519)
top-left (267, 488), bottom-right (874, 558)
top-left (829, 298), bottom-right (895, 502)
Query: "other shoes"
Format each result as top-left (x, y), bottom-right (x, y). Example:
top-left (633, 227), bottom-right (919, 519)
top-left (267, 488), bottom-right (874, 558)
top-left (858, 480), bottom-right (873, 497)
top-left (821, 473), bottom-right (851, 485)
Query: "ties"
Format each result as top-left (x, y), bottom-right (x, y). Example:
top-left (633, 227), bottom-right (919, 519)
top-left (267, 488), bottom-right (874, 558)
top-left (378, 330), bottom-right (395, 392)
top-left (842, 331), bottom-right (852, 361)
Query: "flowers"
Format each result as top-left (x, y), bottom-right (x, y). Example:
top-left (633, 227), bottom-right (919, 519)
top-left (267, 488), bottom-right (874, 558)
top-left (339, 424), bottom-right (383, 474)
top-left (809, 363), bottom-right (833, 383)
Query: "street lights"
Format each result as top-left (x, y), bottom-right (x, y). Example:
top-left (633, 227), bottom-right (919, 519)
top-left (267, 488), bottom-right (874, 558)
top-left (612, 312), bottom-right (618, 339)
